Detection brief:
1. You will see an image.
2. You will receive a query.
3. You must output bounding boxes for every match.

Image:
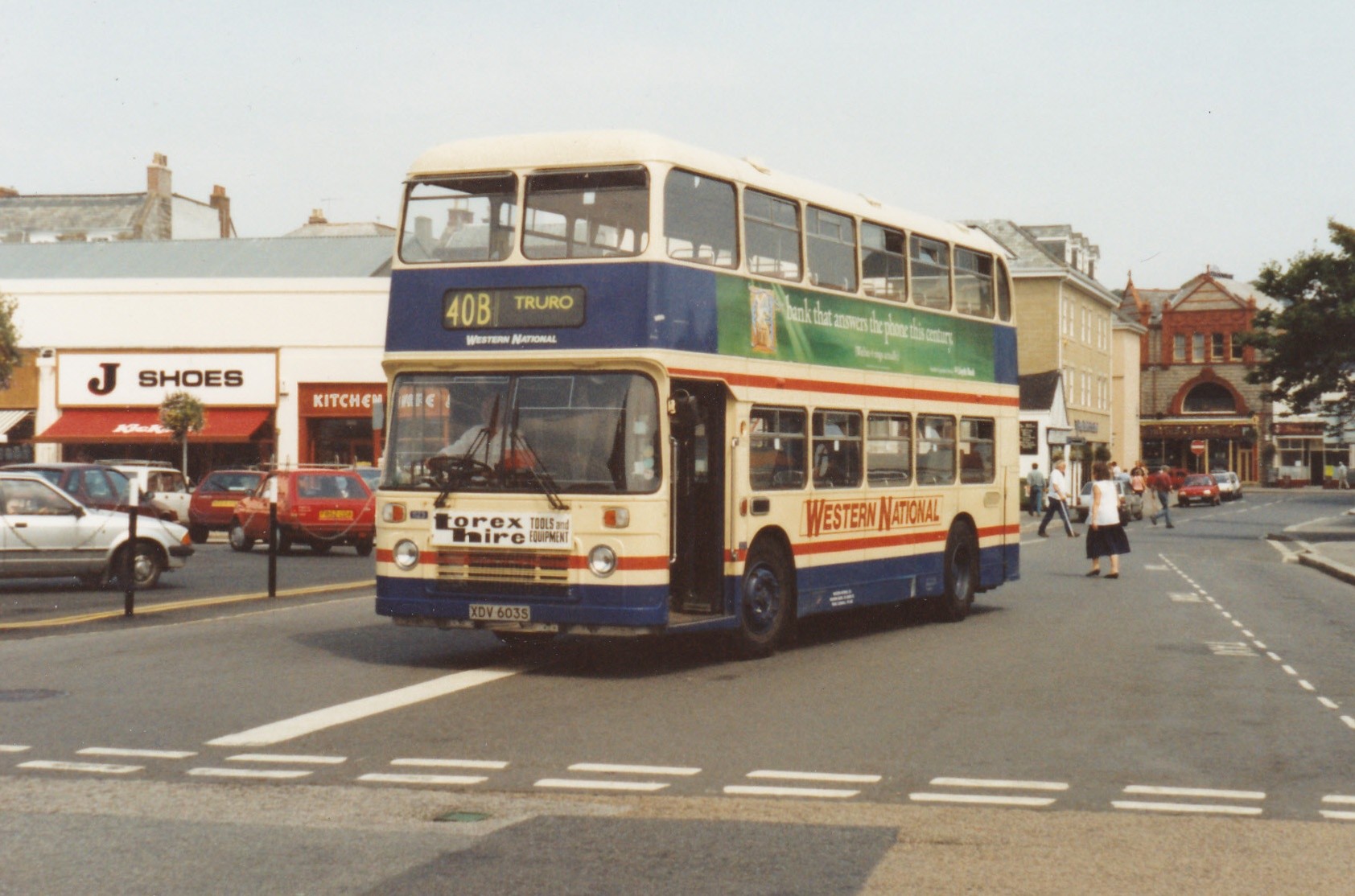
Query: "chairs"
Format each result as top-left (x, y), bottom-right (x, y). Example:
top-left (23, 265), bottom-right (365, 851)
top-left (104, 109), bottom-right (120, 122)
top-left (672, 247), bottom-right (992, 315)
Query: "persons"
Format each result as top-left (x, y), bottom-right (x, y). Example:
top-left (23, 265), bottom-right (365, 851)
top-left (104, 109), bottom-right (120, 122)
top-left (1150, 464), bottom-right (1174, 529)
top-left (1110, 461), bottom-right (1148, 497)
top-left (424, 389), bottom-right (528, 481)
top-left (1338, 461), bottom-right (1349, 490)
top-left (1037, 460), bottom-right (1080, 538)
top-left (1085, 462), bottom-right (1130, 580)
top-left (1027, 462), bottom-right (1046, 515)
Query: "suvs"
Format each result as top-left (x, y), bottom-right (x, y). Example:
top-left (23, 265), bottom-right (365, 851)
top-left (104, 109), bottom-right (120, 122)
top-left (228, 460), bottom-right (377, 555)
top-left (98, 459), bottom-right (192, 527)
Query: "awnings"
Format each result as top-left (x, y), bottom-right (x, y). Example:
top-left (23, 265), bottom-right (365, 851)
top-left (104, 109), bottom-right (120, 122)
top-left (0, 410), bottom-right (30, 443)
top-left (32, 407), bottom-right (270, 443)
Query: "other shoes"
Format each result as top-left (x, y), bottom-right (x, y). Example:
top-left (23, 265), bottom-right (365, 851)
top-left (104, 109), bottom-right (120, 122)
top-left (1166, 524), bottom-right (1174, 529)
top-left (1029, 511), bottom-right (1034, 516)
top-left (1104, 573), bottom-right (1119, 579)
top-left (1038, 532), bottom-right (1049, 538)
top-left (1150, 515), bottom-right (1157, 525)
top-left (1037, 514), bottom-right (1040, 517)
top-left (1068, 532), bottom-right (1081, 537)
top-left (1086, 568), bottom-right (1100, 577)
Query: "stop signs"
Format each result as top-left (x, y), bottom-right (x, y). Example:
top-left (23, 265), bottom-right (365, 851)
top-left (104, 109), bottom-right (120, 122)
top-left (1190, 440), bottom-right (1205, 454)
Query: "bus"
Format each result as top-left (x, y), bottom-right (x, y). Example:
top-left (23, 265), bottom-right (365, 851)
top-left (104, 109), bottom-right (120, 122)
top-left (376, 131), bottom-right (1022, 659)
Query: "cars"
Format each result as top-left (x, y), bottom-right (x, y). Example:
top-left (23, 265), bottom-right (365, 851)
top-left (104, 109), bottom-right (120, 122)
top-left (1210, 472), bottom-right (1243, 501)
top-left (1177, 474), bottom-right (1222, 507)
top-left (337, 464), bottom-right (381, 497)
top-left (1147, 467), bottom-right (1192, 490)
top-left (188, 470), bottom-right (268, 545)
top-left (1076, 482), bottom-right (1144, 526)
top-left (0, 464), bottom-right (180, 524)
top-left (0, 472), bottom-right (195, 593)
